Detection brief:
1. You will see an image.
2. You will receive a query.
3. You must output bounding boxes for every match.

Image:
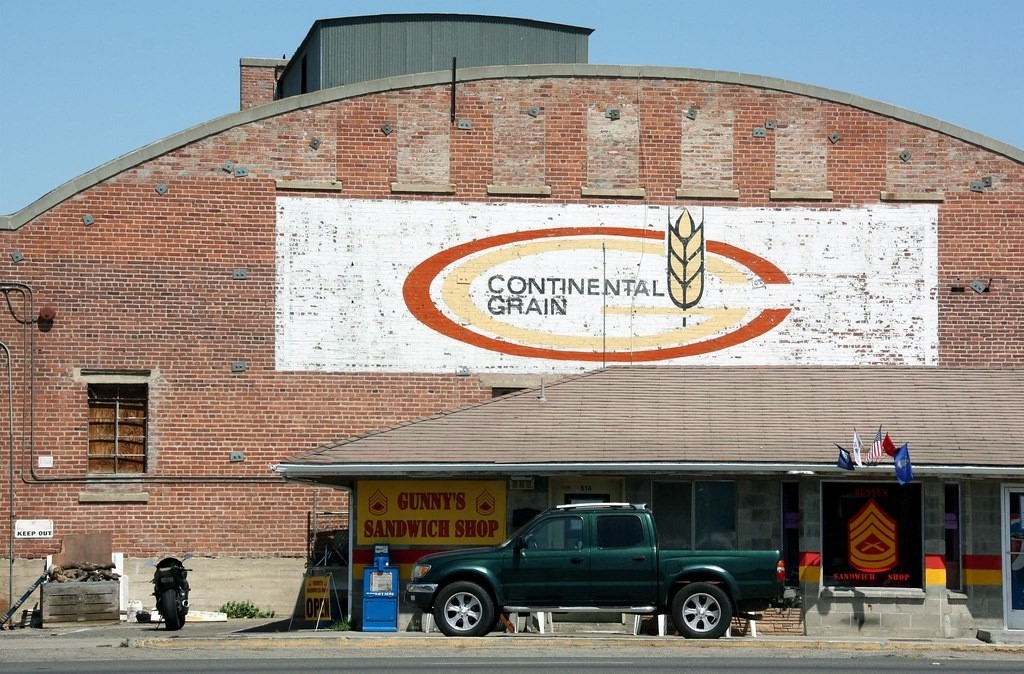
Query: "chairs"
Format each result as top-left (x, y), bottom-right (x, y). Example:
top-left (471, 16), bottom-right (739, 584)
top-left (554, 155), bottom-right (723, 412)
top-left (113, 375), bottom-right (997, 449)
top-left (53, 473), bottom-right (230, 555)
top-left (634, 615), bottom-right (667, 636)
top-left (725, 611), bottom-right (757, 638)
top-left (515, 612), bottom-right (553, 634)
top-left (425, 614), bottom-right (437, 634)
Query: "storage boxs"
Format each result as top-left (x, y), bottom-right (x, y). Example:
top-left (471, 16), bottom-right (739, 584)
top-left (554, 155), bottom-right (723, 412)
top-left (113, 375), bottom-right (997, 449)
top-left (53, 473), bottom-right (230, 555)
top-left (40, 580), bottom-right (120, 627)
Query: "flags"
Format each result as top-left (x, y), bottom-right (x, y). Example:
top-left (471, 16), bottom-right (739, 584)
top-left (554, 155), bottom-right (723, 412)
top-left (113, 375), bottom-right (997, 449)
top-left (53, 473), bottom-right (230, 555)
top-left (837, 446), bottom-right (855, 470)
top-left (866, 428), bottom-right (882, 460)
top-left (882, 434), bottom-right (897, 457)
top-left (894, 442), bottom-right (914, 485)
top-left (853, 431), bottom-right (862, 467)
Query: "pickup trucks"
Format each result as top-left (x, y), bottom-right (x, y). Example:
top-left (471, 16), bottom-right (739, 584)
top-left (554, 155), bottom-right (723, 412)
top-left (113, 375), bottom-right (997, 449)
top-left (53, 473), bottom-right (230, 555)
top-left (403, 503), bottom-right (790, 640)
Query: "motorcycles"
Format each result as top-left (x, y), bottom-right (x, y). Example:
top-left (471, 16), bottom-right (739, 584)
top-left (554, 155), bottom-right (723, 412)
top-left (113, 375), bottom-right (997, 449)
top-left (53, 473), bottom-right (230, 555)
top-left (144, 553), bottom-right (193, 630)
